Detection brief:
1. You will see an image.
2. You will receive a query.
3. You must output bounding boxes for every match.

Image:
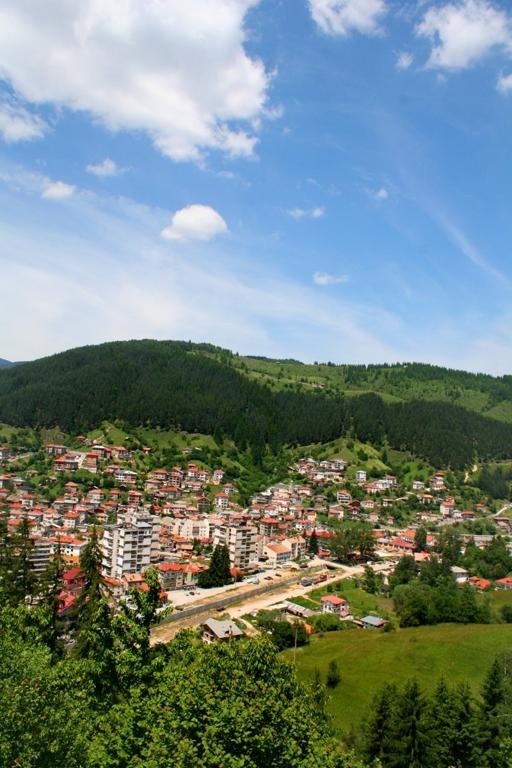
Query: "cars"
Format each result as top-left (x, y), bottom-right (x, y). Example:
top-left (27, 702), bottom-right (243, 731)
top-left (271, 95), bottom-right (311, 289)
top-left (185, 591), bottom-right (201, 596)
top-left (301, 574), bottom-right (335, 587)
top-left (247, 578), bottom-right (260, 584)
